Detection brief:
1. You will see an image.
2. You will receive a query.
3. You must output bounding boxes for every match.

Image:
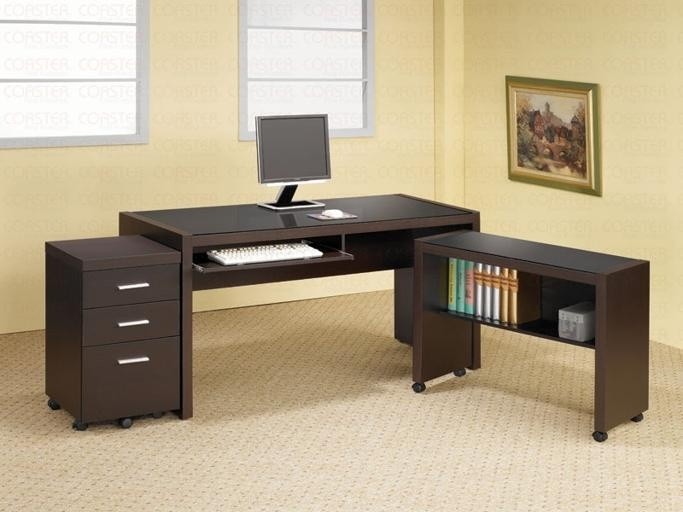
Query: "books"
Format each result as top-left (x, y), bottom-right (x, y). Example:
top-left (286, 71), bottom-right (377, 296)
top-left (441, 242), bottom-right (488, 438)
top-left (446, 257), bottom-right (542, 326)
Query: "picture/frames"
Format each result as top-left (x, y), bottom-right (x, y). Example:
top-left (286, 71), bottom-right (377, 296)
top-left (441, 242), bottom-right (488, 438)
top-left (505, 75), bottom-right (604, 198)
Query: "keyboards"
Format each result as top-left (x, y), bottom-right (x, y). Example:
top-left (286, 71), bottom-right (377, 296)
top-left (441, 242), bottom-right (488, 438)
top-left (205, 242), bottom-right (324, 266)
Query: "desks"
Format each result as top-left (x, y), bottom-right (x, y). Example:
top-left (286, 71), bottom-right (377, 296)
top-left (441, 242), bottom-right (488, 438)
top-left (117, 194), bottom-right (481, 420)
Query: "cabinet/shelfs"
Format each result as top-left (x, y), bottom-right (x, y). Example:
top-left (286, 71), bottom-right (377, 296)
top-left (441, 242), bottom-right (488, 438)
top-left (413, 229), bottom-right (650, 442)
top-left (45, 236), bottom-right (181, 431)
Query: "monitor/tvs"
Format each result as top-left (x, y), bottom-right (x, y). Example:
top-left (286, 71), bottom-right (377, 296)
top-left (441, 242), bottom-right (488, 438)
top-left (254, 113), bottom-right (332, 211)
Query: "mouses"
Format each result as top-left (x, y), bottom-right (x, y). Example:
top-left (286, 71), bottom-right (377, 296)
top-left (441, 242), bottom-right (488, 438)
top-left (322, 209), bottom-right (343, 219)
